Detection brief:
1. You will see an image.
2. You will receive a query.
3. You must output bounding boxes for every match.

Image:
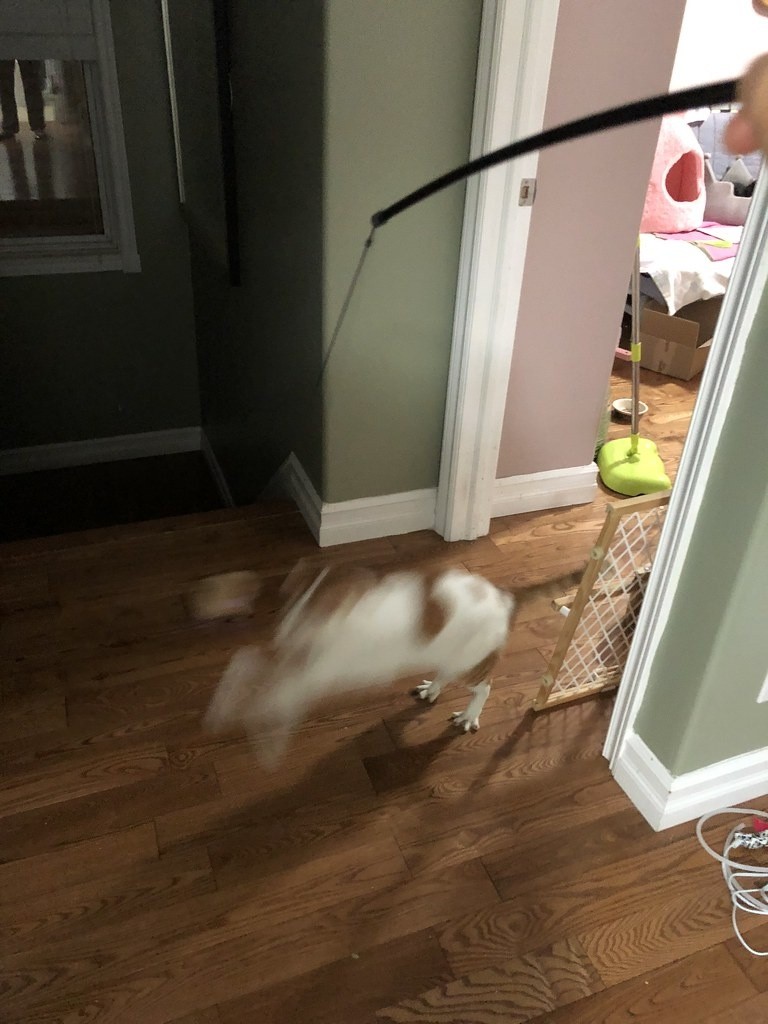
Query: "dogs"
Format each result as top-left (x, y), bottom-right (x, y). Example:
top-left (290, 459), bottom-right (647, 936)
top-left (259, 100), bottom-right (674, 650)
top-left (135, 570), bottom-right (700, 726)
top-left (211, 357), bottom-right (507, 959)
top-left (206, 559), bottom-right (515, 764)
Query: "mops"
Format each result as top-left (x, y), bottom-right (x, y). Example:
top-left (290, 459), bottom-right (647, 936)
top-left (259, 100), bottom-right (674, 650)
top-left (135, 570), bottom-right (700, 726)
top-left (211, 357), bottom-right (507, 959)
top-left (598, 238), bottom-right (674, 496)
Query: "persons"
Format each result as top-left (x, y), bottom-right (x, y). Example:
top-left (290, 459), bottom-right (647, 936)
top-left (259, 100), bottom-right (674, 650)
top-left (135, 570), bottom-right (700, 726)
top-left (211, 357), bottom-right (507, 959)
top-left (0, 59), bottom-right (52, 142)
top-left (720, 50), bottom-right (768, 164)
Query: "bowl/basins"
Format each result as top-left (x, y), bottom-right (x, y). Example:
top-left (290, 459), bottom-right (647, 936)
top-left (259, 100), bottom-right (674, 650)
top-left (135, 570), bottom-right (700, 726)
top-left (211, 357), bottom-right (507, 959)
top-left (612, 399), bottom-right (648, 424)
